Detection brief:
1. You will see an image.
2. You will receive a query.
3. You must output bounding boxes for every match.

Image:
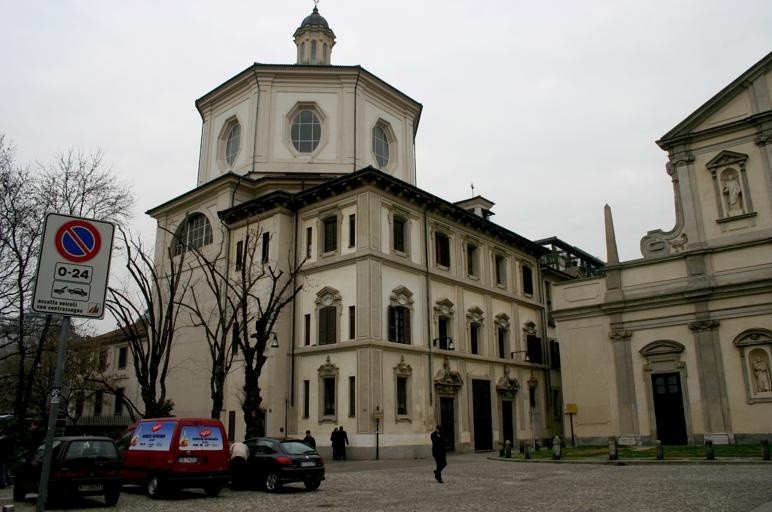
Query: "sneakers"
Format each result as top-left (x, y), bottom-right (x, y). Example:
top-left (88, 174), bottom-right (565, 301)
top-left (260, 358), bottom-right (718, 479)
top-left (433, 470), bottom-right (443, 483)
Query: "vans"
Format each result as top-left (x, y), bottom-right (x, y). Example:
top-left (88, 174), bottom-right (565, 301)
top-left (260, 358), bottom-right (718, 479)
top-left (109, 417), bottom-right (233, 502)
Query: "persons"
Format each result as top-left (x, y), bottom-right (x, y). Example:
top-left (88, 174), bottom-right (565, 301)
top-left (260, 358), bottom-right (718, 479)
top-left (722, 173), bottom-right (743, 211)
top-left (430, 424), bottom-right (449, 483)
top-left (330, 426), bottom-right (340, 461)
top-left (302, 429), bottom-right (317, 451)
top-left (228, 439), bottom-right (250, 492)
top-left (334, 426), bottom-right (350, 461)
top-left (28, 420), bottom-right (45, 459)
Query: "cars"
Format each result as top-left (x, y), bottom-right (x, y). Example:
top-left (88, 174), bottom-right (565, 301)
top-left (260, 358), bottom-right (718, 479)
top-left (227, 436), bottom-right (327, 492)
top-left (12, 435), bottom-right (123, 506)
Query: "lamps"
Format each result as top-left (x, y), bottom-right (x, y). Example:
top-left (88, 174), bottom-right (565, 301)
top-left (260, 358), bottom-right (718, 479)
top-left (433, 337), bottom-right (455, 350)
top-left (250, 331), bottom-right (279, 348)
top-left (511, 350), bottom-right (531, 362)
